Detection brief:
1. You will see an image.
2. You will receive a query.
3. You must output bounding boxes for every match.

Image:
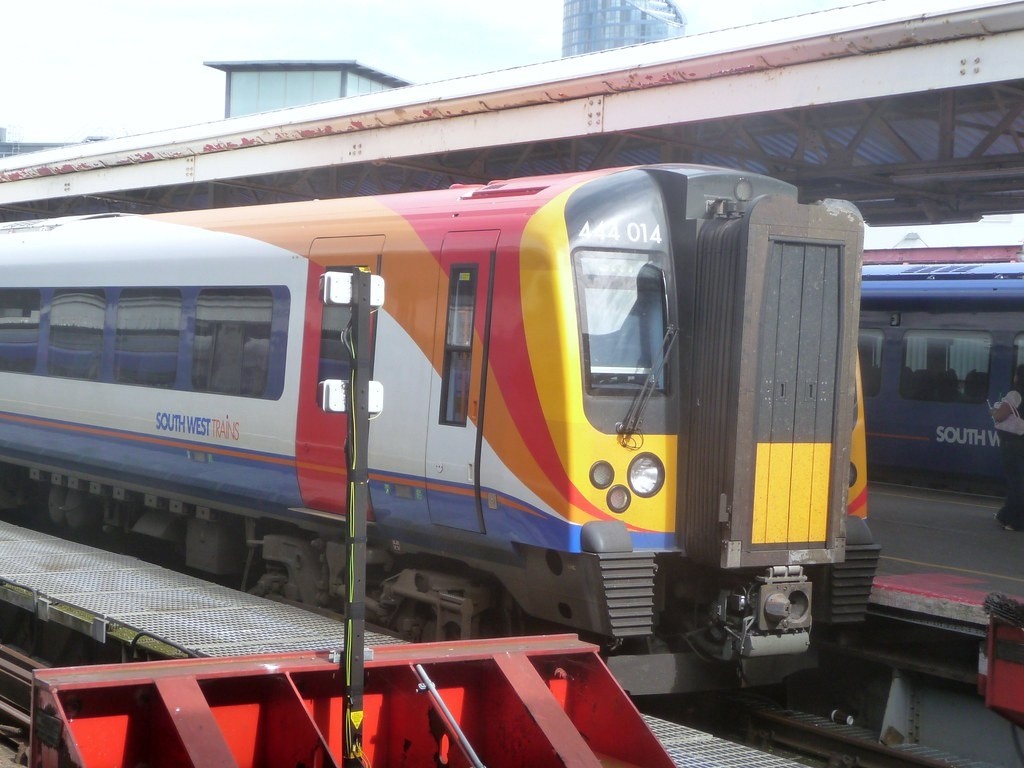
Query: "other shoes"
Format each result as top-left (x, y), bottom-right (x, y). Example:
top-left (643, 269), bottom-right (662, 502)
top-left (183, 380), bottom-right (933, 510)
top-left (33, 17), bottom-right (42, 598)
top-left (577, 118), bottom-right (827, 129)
top-left (1004, 524), bottom-right (1024, 531)
top-left (994, 513), bottom-right (1010, 523)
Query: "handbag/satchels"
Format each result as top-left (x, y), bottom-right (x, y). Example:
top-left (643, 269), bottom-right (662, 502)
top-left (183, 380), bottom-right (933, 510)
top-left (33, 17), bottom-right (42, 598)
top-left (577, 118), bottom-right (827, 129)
top-left (992, 397), bottom-right (1024, 435)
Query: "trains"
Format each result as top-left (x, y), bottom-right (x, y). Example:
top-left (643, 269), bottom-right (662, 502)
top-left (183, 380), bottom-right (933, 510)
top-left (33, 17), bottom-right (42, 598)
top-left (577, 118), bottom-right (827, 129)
top-left (851, 264), bottom-right (1024, 487)
top-left (1, 163), bottom-right (882, 719)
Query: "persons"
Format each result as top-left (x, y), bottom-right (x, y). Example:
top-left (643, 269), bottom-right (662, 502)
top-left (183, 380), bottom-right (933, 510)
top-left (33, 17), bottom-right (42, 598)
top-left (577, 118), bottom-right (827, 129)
top-left (987, 363), bottom-right (1024, 532)
